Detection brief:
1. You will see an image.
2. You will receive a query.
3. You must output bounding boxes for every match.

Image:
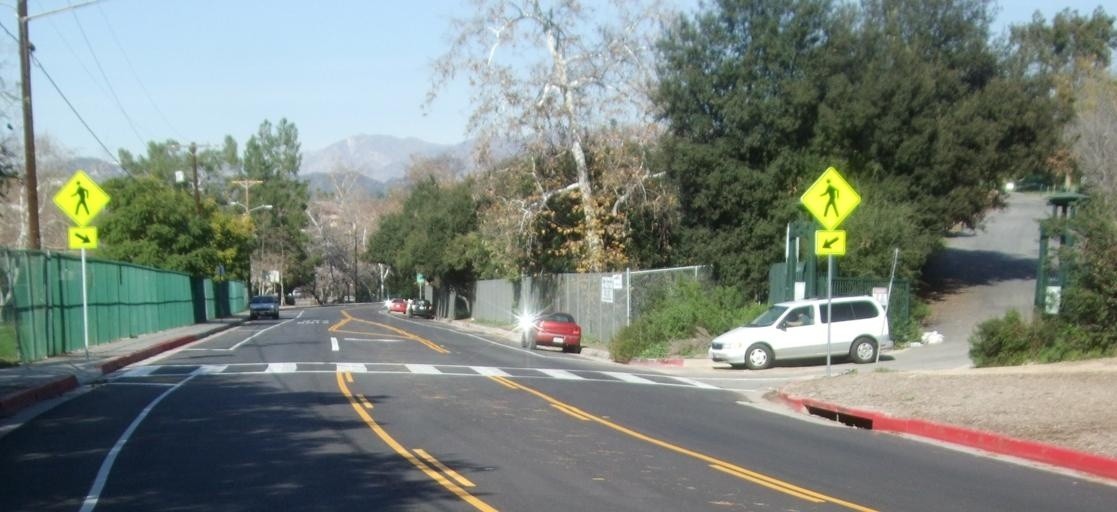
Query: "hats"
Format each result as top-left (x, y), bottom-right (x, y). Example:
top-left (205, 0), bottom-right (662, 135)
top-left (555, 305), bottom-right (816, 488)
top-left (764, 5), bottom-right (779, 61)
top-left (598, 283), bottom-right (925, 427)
top-left (388, 298), bottom-right (407, 314)
top-left (701, 291), bottom-right (896, 374)
top-left (407, 297), bottom-right (436, 318)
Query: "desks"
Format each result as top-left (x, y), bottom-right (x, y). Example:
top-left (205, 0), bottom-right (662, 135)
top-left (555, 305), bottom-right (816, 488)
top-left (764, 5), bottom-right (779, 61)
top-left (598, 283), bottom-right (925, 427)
top-left (227, 199), bottom-right (273, 215)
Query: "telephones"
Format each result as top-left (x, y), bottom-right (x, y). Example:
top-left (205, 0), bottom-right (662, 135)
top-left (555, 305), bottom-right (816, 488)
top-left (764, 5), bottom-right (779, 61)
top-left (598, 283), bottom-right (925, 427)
top-left (416, 271), bottom-right (425, 286)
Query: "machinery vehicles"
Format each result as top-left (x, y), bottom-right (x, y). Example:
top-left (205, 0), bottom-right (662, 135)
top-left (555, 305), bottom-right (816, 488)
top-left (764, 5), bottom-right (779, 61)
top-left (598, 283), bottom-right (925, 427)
top-left (517, 311), bottom-right (583, 357)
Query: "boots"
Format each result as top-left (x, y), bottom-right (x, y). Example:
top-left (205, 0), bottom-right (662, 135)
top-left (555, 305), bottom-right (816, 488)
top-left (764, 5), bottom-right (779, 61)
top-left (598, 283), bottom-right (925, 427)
top-left (249, 294), bottom-right (273, 321)
